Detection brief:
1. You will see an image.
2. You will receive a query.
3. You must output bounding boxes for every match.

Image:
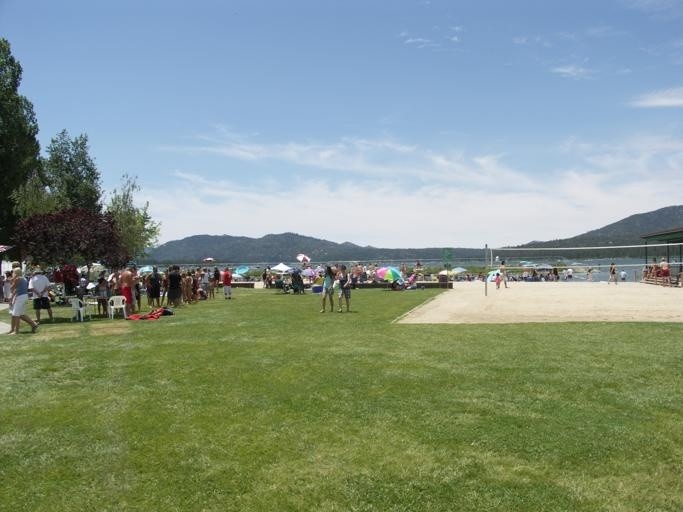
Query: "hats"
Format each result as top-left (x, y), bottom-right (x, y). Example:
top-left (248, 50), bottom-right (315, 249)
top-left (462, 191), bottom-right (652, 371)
top-left (33, 268), bottom-right (44, 273)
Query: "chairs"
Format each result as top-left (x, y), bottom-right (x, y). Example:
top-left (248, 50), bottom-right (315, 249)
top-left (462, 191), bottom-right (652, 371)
top-left (68, 295), bottom-right (128, 321)
